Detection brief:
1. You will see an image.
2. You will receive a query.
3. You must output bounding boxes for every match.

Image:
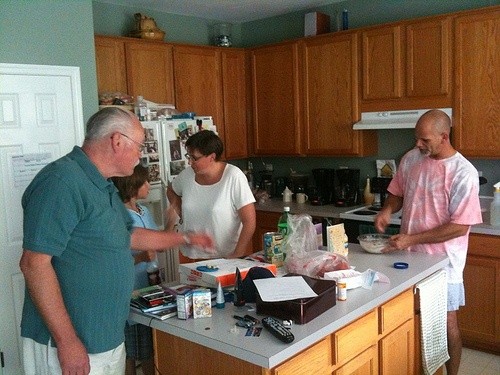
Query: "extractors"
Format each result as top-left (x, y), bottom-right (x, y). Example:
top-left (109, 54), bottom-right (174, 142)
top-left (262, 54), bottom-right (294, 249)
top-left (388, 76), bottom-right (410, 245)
top-left (352, 108), bottom-right (453, 131)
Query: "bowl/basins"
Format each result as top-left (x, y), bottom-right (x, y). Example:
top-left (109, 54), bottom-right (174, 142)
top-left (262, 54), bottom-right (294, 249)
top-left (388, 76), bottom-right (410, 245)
top-left (357, 234), bottom-right (392, 254)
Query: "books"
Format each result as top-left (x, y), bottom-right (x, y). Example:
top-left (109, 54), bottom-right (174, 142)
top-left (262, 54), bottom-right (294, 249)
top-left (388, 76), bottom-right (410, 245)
top-left (127, 285), bottom-right (178, 322)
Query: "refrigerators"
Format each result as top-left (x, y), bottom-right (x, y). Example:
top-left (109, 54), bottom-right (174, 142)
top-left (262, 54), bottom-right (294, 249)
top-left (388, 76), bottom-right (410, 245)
top-left (134, 116), bottom-right (220, 288)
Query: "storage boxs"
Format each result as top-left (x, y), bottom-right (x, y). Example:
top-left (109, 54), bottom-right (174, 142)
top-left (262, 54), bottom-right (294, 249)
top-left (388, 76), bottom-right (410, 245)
top-left (176, 258), bottom-right (278, 319)
top-left (304, 12), bottom-right (330, 38)
top-left (256, 272), bottom-right (337, 324)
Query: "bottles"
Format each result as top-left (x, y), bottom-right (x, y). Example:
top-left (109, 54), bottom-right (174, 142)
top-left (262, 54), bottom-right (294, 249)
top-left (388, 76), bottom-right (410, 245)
top-left (363, 174), bottom-right (375, 204)
top-left (277, 206), bottom-right (291, 240)
top-left (147, 260), bottom-right (162, 285)
top-left (337, 279), bottom-right (348, 302)
top-left (134, 95), bottom-right (147, 121)
top-left (344, 233), bottom-right (349, 256)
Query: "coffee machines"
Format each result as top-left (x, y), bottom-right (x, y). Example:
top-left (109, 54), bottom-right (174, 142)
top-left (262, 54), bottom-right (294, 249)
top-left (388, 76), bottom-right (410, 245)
top-left (335, 168), bottom-right (362, 207)
top-left (310, 168), bottom-right (335, 205)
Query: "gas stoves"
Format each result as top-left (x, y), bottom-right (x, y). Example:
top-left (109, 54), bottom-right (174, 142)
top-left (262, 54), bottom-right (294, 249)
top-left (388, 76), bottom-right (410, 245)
top-left (339, 178), bottom-right (403, 225)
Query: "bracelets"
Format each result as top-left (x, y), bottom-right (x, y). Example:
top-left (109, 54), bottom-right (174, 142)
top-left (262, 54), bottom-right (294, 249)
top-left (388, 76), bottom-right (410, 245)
top-left (182, 231), bottom-right (193, 248)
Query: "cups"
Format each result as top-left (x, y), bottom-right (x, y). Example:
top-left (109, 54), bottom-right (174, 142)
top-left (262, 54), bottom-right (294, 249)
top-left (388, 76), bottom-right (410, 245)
top-left (296, 193), bottom-right (308, 203)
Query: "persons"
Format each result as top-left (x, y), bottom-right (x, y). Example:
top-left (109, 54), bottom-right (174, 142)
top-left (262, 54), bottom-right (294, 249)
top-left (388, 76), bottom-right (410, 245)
top-left (18, 107), bottom-right (214, 375)
top-left (166, 130), bottom-right (257, 261)
top-left (112, 164), bottom-right (179, 375)
top-left (375, 110), bottom-right (484, 375)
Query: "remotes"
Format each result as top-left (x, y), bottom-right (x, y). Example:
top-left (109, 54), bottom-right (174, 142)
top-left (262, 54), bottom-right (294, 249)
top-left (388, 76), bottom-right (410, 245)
top-left (262, 316), bottom-right (294, 344)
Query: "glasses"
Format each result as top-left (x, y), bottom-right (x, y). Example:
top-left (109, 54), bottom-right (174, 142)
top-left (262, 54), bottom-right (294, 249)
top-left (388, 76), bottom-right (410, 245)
top-left (184, 153), bottom-right (207, 162)
top-left (111, 133), bottom-right (146, 152)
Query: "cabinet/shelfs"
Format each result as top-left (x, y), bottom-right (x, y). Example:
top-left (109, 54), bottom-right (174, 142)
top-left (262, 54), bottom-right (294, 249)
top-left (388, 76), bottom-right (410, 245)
top-left (456, 234), bottom-right (500, 356)
top-left (356, 12), bottom-right (452, 112)
top-left (151, 287), bottom-right (444, 375)
top-left (246, 29), bottom-right (379, 158)
top-left (244, 211), bottom-right (281, 256)
top-left (453, 5), bottom-right (500, 162)
top-left (96, 35), bottom-right (250, 160)
